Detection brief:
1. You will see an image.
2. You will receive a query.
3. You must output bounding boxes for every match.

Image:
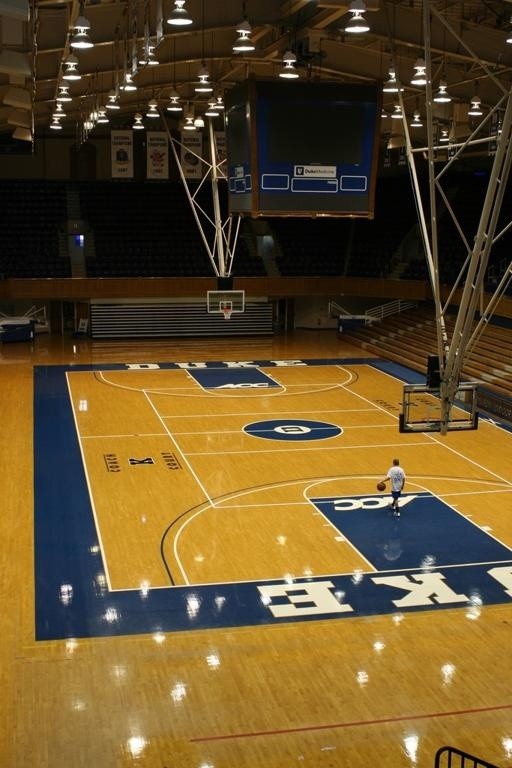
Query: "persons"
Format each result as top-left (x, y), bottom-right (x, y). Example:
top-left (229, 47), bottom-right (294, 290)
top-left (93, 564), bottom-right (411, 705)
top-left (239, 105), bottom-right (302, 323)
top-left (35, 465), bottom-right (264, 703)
top-left (379, 458), bottom-right (405, 517)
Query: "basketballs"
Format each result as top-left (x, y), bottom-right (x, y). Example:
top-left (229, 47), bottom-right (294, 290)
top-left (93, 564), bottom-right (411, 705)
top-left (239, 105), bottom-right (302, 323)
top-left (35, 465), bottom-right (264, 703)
top-left (377, 482), bottom-right (385, 491)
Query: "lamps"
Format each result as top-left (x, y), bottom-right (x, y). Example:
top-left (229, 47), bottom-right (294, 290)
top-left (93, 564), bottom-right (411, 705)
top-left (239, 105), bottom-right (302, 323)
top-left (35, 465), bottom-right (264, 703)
top-left (279, 4), bottom-right (299, 78)
top-left (195, 2), bottom-right (224, 117)
top-left (165, 35), bottom-right (204, 130)
top-left (344, 1), bottom-right (370, 33)
top-left (377, 2), bottom-right (485, 147)
top-left (48, 2), bottom-right (164, 130)
top-left (233, 2), bottom-right (255, 51)
top-left (167, 1), bottom-right (193, 25)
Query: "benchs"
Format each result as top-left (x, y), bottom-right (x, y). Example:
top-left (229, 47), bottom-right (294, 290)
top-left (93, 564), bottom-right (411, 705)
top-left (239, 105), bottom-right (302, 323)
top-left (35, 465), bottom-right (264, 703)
top-left (332, 299), bottom-right (512, 401)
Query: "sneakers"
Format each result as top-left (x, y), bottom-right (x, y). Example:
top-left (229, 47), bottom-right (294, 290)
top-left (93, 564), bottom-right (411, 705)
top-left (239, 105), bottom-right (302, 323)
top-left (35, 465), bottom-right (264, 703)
top-left (388, 502), bottom-right (395, 509)
top-left (394, 511), bottom-right (400, 517)
